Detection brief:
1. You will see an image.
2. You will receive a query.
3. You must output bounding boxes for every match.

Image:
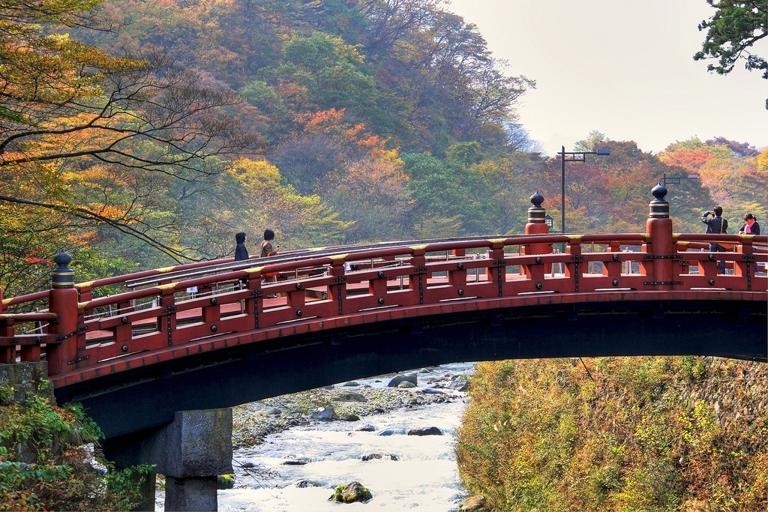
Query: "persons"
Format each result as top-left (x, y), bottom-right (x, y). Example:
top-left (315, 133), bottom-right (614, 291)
top-left (700, 205), bottom-right (728, 275)
top-left (233, 232), bottom-right (249, 301)
top-left (260, 229), bottom-right (280, 298)
top-left (737, 213), bottom-right (760, 275)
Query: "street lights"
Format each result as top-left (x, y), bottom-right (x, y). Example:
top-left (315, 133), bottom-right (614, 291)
top-left (659, 171), bottom-right (698, 187)
top-left (557, 145), bottom-right (613, 272)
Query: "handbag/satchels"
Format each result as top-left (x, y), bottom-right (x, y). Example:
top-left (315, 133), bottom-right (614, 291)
top-left (718, 232), bottom-right (736, 249)
